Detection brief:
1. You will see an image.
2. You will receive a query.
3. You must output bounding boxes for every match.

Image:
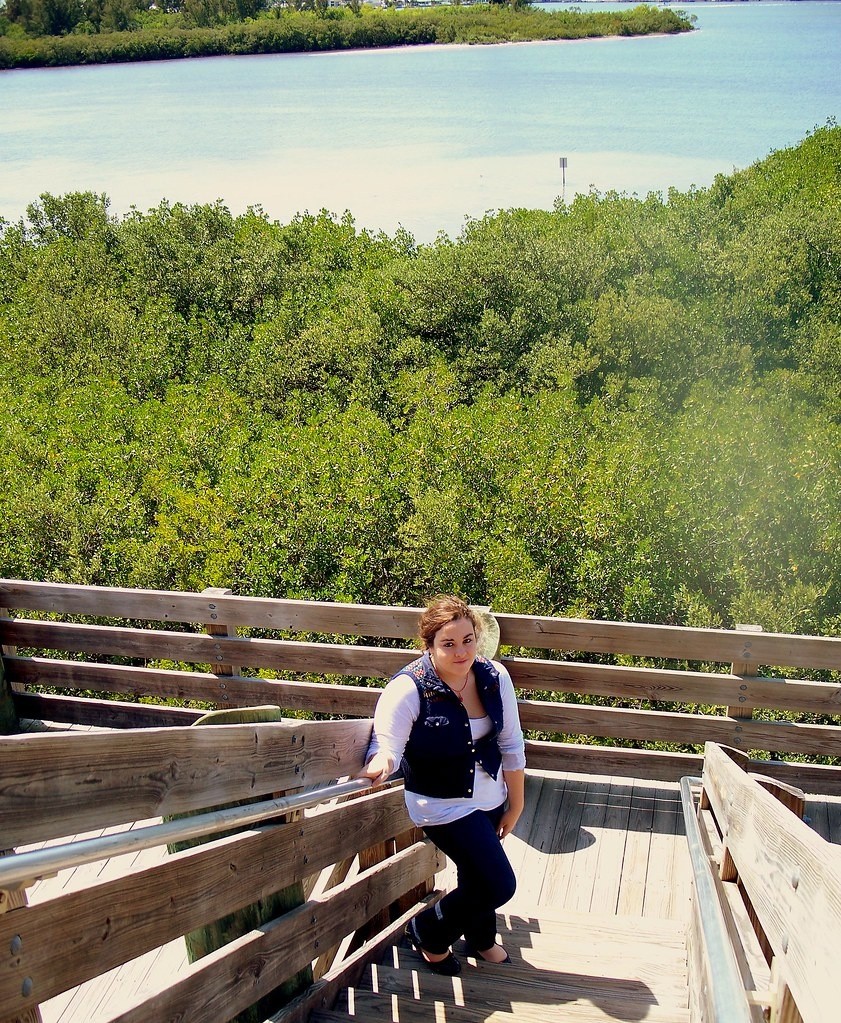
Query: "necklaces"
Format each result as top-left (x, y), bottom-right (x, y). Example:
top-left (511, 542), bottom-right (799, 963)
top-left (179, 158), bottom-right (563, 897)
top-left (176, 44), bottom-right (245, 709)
top-left (432, 662), bottom-right (470, 704)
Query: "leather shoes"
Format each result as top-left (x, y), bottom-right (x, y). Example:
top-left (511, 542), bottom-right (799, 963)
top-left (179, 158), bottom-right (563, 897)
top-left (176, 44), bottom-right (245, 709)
top-left (405, 924), bottom-right (461, 976)
top-left (464, 940), bottom-right (512, 964)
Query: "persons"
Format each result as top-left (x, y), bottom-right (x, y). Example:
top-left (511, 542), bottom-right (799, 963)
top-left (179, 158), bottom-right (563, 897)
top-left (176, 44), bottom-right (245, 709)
top-left (350, 592), bottom-right (528, 978)
